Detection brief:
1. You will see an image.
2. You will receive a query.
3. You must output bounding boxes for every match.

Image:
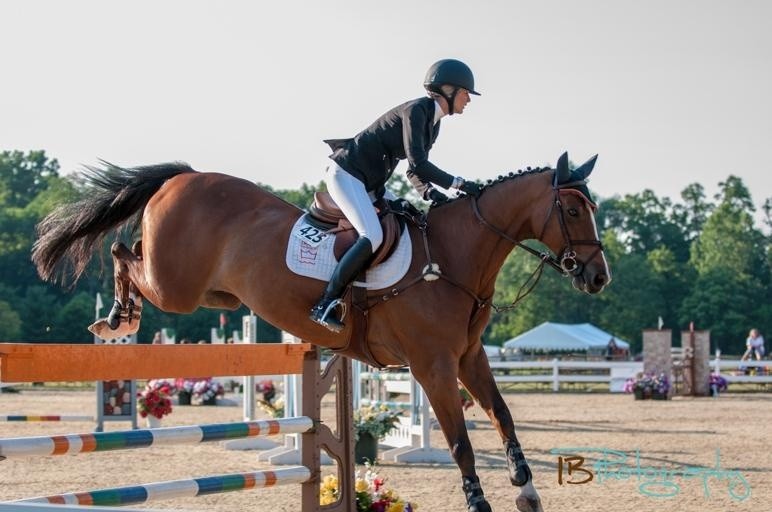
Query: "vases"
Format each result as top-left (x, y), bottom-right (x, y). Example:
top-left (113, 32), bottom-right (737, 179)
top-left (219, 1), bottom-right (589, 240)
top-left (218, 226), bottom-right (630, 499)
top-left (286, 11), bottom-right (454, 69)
top-left (634, 388), bottom-right (646, 399)
top-left (652, 390), bottom-right (667, 399)
top-left (354, 429), bottom-right (378, 467)
top-left (711, 387), bottom-right (719, 397)
top-left (147, 412), bottom-right (162, 428)
top-left (178, 392), bottom-right (190, 405)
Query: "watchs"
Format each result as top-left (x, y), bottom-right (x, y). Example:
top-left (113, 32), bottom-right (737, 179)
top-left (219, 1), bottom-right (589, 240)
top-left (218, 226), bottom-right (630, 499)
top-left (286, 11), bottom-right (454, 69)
top-left (457, 177), bottom-right (463, 189)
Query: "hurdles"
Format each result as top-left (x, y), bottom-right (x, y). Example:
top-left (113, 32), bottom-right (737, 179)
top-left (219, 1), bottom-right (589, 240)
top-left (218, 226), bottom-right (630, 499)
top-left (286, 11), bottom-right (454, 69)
top-left (0, 292), bottom-right (474, 512)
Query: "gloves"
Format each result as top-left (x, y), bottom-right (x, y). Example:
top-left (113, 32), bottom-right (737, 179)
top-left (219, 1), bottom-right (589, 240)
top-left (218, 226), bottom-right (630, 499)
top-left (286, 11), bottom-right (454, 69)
top-left (428, 188), bottom-right (451, 203)
top-left (459, 182), bottom-right (484, 196)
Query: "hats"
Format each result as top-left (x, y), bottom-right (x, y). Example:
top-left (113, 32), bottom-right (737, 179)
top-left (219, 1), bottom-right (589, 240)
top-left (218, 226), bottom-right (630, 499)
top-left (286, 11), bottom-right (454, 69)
top-left (423, 60), bottom-right (483, 96)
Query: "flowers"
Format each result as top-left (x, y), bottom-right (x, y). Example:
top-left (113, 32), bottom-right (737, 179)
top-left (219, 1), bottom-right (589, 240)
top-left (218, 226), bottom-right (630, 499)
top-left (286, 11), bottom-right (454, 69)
top-left (350, 400), bottom-right (408, 438)
top-left (177, 377), bottom-right (194, 394)
top-left (136, 380), bottom-right (174, 419)
top-left (710, 374), bottom-right (728, 392)
top-left (624, 372), bottom-right (670, 394)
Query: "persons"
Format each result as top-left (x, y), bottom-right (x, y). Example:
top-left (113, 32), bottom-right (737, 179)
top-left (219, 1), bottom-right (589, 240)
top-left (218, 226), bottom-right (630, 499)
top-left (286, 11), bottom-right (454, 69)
top-left (310, 59), bottom-right (485, 333)
top-left (741, 329), bottom-right (766, 370)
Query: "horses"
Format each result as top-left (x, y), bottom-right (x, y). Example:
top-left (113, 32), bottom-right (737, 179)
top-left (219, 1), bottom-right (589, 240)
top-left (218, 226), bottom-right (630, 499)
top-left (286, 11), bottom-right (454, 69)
top-left (29, 150), bottom-right (610, 512)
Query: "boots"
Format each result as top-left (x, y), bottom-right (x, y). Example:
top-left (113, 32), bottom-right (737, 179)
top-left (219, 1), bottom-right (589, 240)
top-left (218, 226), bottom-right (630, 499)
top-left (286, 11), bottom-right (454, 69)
top-left (309, 235), bottom-right (372, 331)
